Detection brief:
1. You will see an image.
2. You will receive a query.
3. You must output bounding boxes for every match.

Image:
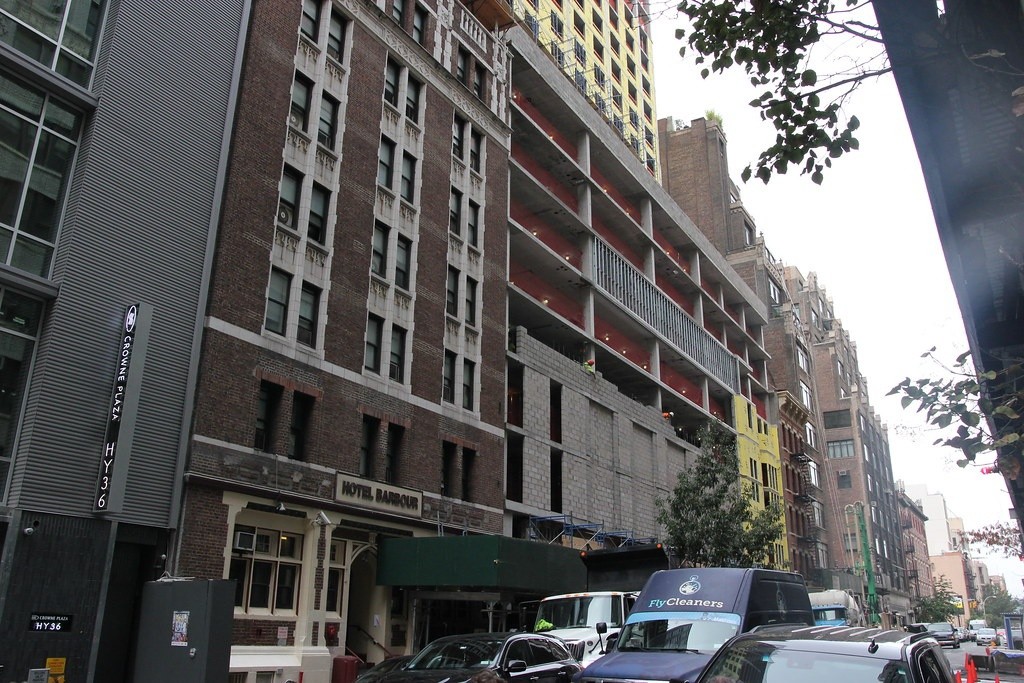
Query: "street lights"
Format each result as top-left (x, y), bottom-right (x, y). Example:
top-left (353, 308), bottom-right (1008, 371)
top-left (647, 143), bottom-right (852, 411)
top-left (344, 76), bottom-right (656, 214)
top-left (983, 595), bottom-right (996, 622)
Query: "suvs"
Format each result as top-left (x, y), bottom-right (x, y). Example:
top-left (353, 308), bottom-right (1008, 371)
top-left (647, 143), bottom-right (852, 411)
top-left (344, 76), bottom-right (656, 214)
top-left (926, 623), bottom-right (960, 649)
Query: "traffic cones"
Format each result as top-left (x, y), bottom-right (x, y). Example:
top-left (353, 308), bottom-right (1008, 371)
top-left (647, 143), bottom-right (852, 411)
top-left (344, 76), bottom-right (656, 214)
top-left (971, 659), bottom-right (977, 682)
top-left (967, 665), bottom-right (974, 683)
top-left (995, 675), bottom-right (1000, 683)
top-left (964, 653), bottom-right (969, 671)
top-left (956, 671), bottom-right (962, 683)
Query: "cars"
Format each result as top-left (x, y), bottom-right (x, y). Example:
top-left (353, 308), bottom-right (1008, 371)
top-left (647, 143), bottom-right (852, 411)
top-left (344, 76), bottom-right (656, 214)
top-left (956, 627), bottom-right (969, 642)
top-left (692, 622), bottom-right (956, 683)
top-left (356, 631), bottom-right (586, 683)
top-left (977, 628), bottom-right (1001, 646)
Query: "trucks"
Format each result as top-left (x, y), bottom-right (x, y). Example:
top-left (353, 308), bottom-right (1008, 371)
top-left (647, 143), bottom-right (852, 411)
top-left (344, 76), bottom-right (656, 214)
top-left (969, 620), bottom-right (989, 642)
top-left (532, 590), bottom-right (641, 671)
top-left (570, 568), bottom-right (816, 683)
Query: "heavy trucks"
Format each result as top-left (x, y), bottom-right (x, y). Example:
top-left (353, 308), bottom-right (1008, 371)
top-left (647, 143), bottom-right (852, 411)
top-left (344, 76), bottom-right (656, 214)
top-left (803, 590), bottom-right (867, 629)
